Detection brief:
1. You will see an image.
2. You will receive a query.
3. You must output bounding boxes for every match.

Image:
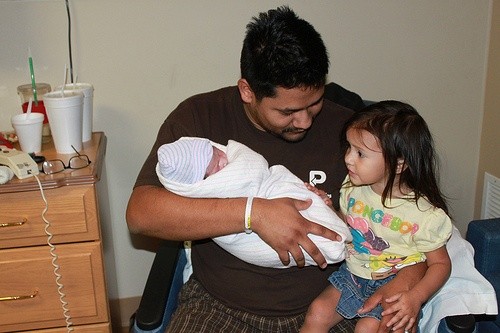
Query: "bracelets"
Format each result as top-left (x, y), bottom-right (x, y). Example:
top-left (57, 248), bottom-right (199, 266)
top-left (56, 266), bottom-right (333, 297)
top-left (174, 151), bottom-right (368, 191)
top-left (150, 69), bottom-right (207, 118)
top-left (243, 194), bottom-right (256, 236)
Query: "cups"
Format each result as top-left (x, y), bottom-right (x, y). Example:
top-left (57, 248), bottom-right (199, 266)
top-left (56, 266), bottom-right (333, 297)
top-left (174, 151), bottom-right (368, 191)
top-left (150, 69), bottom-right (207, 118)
top-left (11, 112), bottom-right (44, 153)
top-left (41, 90), bottom-right (84, 154)
top-left (54, 83), bottom-right (94, 143)
top-left (16, 83), bottom-right (52, 137)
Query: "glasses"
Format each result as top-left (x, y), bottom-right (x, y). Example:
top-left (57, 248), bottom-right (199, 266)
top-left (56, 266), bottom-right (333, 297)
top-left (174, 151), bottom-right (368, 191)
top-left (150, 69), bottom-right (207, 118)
top-left (39, 145), bottom-right (92, 175)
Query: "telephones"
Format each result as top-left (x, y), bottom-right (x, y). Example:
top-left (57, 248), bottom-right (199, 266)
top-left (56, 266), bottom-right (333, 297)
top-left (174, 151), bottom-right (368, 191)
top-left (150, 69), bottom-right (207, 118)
top-left (0, 145), bottom-right (40, 180)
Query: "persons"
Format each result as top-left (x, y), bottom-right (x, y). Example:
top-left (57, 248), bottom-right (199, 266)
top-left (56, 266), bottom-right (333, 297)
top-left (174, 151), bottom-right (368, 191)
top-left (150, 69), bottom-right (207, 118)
top-left (122, 0), bottom-right (430, 333)
top-left (156, 135), bottom-right (352, 269)
top-left (299, 98), bottom-right (453, 333)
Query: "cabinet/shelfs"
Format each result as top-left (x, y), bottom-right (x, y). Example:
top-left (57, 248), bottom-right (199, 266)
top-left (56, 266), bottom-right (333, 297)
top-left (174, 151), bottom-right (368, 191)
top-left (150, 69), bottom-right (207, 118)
top-left (1, 131), bottom-right (112, 333)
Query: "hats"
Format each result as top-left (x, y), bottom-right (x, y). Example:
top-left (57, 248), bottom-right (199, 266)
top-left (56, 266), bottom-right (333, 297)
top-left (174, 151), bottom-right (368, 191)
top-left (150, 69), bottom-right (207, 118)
top-left (157, 136), bottom-right (213, 184)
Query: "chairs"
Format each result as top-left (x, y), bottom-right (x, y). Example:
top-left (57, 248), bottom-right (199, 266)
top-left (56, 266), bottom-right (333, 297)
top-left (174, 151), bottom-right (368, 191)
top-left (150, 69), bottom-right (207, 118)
top-left (127, 238), bottom-right (475, 333)
